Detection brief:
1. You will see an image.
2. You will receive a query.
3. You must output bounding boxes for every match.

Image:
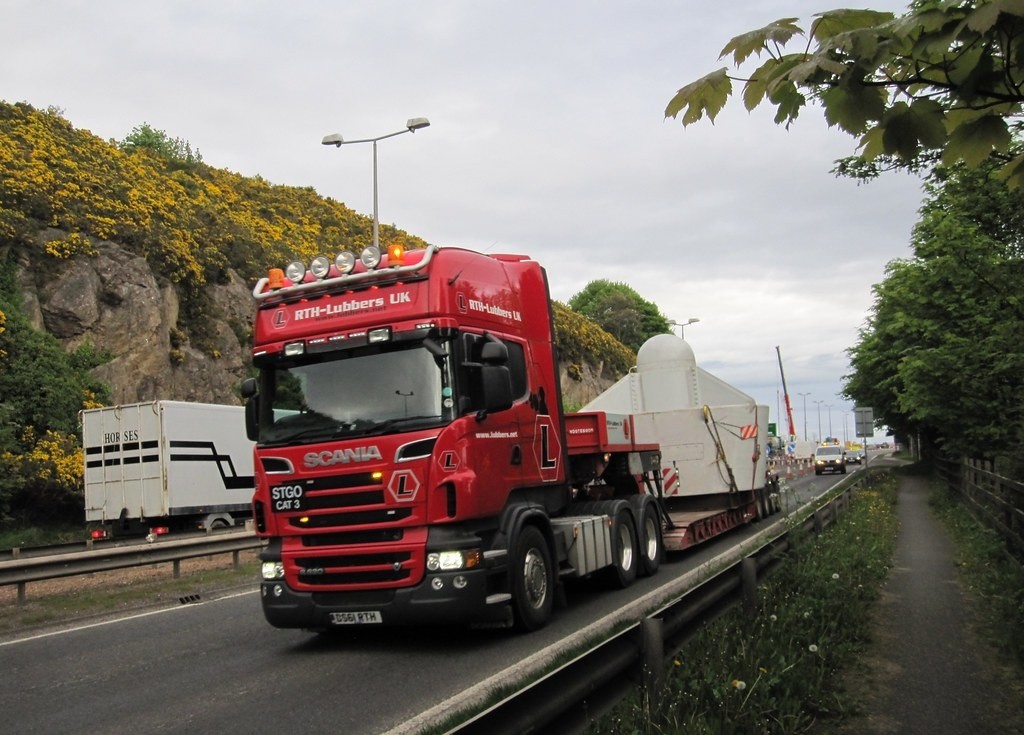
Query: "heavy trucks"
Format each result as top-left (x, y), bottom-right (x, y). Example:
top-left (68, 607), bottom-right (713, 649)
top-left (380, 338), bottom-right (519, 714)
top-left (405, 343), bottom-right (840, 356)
top-left (768, 436), bottom-right (784, 456)
top-left (238, 243), bottom-right (780, 639)
top-left (81, 401), bottom-right (306, 543)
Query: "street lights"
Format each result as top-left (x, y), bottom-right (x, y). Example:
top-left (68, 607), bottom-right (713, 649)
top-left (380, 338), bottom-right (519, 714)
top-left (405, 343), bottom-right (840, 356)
top-left (824, 404), bottom-right (833, 442)
top-left (813, 399), bottom-right (823, 443)
top-left (322, 118), bottom-right (431, 248)
top-left (663, 319), bottom-right (700, 339)
top-left (843, 410), bottom-right (850, 443)
top-left (798, 392), bottom-right (812, 441)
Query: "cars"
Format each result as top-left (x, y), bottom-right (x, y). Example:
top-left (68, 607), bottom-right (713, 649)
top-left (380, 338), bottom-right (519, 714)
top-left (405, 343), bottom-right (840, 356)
top-left (844, 450), bottom-right (861, 465)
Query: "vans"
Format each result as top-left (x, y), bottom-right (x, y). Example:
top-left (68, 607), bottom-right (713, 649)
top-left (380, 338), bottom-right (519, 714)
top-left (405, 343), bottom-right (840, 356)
top-left (813, 445), bottom-right (847, 475)
